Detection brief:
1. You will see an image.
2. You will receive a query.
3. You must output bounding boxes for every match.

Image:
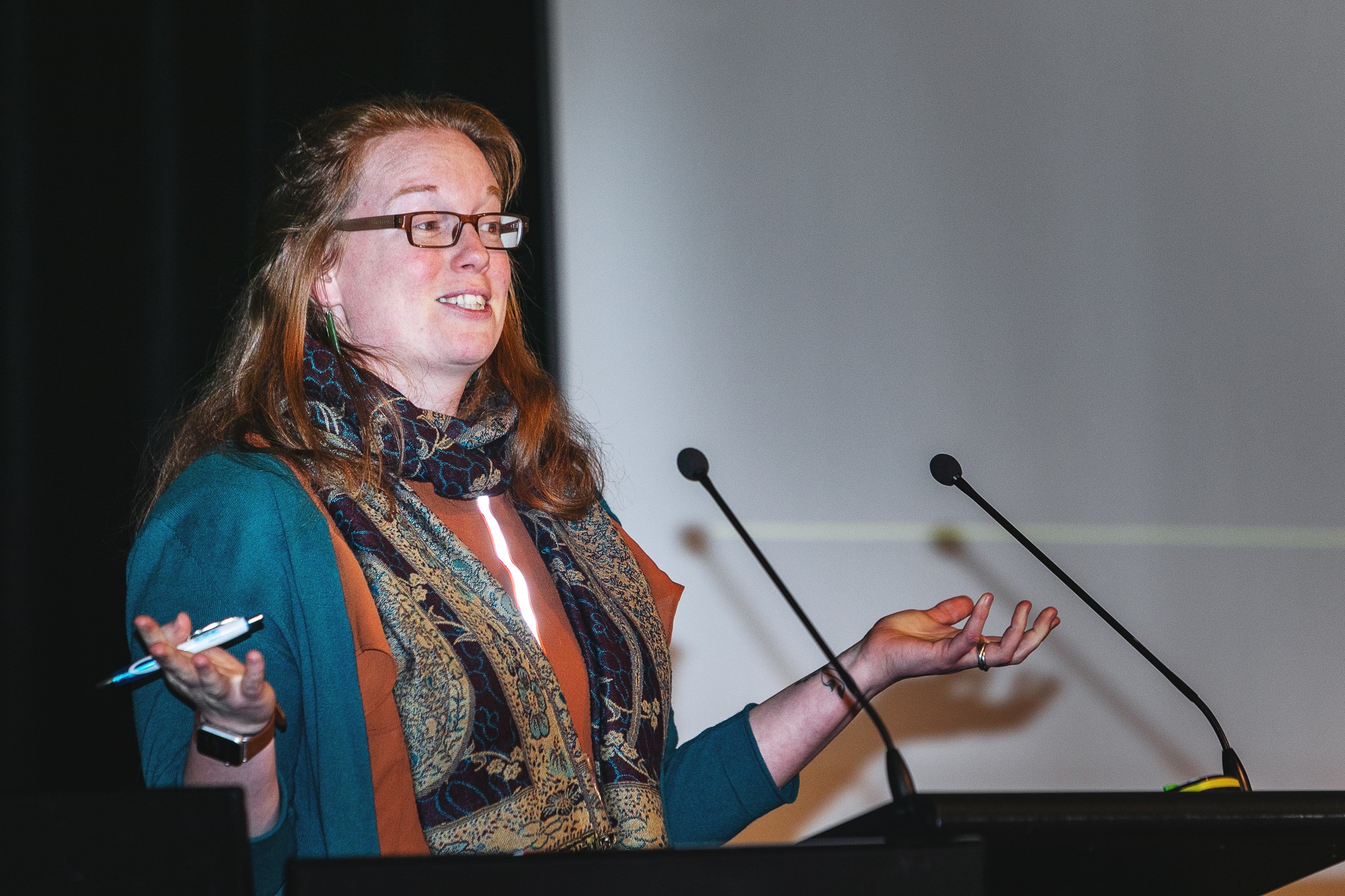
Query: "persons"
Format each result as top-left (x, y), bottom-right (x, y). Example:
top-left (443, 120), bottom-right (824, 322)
top-left (123, 94), bottom-right (1061, 895)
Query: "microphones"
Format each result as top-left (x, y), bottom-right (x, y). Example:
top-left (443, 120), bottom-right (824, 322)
top-left (930, 453), bottom-right (1256, 796)
top-left (678, 447), bottom-right (912, 797)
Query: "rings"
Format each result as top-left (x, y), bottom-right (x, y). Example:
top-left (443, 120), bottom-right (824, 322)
top-left (976, 639), bottom-right (991, 672)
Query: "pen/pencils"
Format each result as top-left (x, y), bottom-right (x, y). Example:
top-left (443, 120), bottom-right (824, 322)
top-left (104, 611), bottom-right (266, 682)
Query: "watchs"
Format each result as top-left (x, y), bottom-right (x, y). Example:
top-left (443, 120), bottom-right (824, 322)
top-left (193, 702), bottom-right (288, 767)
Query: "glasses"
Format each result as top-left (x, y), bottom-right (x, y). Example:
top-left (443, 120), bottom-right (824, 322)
top-left (333, 211), bottom-right (529, 249)
